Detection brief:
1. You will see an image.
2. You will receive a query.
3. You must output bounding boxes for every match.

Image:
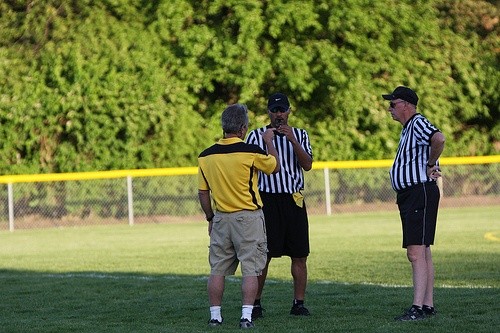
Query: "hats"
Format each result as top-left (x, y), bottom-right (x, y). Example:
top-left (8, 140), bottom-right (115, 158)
top-left (268, 94), bottom-right (290, 111)
top-left (382, 86), bottom-right (419, 106)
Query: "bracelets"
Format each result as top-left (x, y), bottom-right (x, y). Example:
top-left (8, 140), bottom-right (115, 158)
top-left (206, 214), bottom-right (215, 222)
top-left (427, 161), bottom-right (436, 168)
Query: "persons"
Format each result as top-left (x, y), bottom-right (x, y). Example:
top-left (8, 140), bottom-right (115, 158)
top-left (381, 86), bottom-right (444, 321)
top-left (196, 105), bottom-right (281, 331)
top-left (244, 94), bottom-right (313, 321)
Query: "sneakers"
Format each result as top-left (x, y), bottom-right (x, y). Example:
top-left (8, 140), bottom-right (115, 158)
top-left (240, 319), bottom-right (255, 327)
top-left (252, 306), bottom-right (263, 320)
top-left (394, 309), bottom-right (424, 320)
top-left (290, 304), bottom-right (310, 315)
top-left (421, 311), bottom-right (436, 319)
top-left (209, 317), bottom-right (224, 325)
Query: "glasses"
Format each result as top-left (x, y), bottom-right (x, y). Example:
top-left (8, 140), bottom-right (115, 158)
top-left (390, 100), bottom-right (404, 108)
top-left (270, 107), bottom-right (287, 113)
